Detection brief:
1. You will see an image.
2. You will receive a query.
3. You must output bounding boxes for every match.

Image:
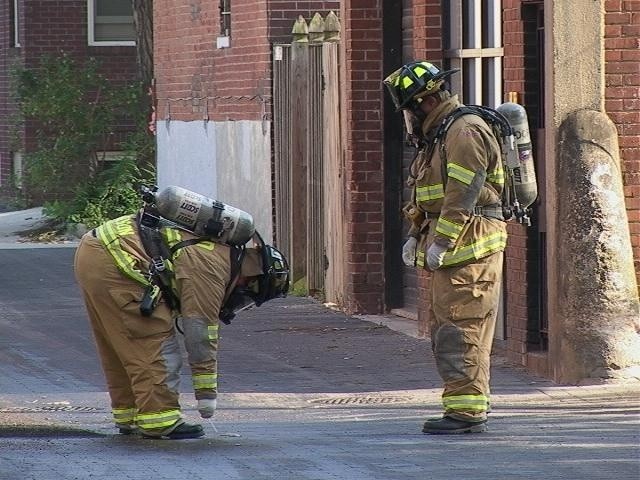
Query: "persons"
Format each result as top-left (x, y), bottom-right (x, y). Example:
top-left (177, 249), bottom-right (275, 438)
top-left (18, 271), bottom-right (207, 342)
top-left (74, 181), bottom-right (291, 440)
top-left (383, 61), bottom-right (539, 435)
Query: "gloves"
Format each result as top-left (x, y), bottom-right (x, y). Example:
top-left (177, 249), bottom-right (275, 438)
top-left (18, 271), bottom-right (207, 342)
top-left (198, 398), bottom-right (216, 419)
top-left (427, 243), bottom-right (447, 272)
top-left (402, 237), bottom-right (417, 268)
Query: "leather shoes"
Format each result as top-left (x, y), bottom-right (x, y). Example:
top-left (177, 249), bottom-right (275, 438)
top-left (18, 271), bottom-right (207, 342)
top-left (423, 416), bottom-right (486, 436)
top-left (118, 419), bottom-right (203, 440)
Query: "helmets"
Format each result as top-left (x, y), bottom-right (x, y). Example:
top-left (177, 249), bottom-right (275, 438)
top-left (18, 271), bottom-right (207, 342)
top-left (260, 245), bottom-right (290, 306)
top-left (394, 61), bottom-right (461, 112)
